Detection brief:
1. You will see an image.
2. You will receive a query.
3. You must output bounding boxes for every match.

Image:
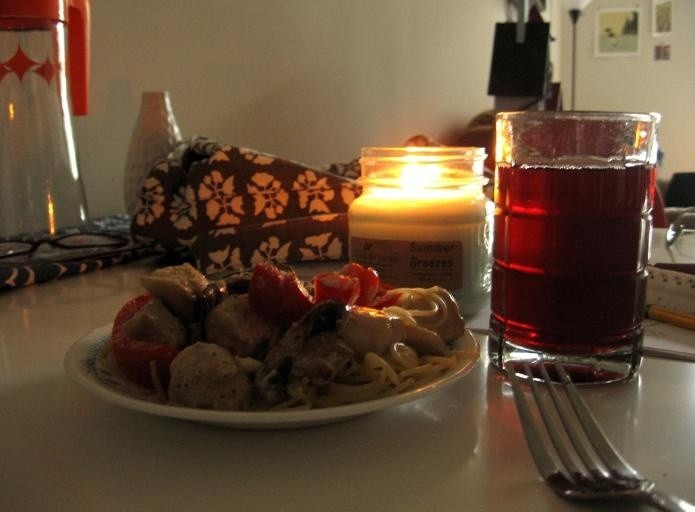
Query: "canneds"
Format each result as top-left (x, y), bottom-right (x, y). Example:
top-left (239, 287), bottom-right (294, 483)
top-left (348, 146), bottom-right (489, 316)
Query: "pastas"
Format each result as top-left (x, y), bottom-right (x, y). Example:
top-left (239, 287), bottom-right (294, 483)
top-left (151, 284), bottom-right (480, 409)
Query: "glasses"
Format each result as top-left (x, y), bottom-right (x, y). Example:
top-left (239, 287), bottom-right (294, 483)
top-left (0, 231), bottom-right (128, 259)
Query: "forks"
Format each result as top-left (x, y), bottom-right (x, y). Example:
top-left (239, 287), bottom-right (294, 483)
top-left (502, 359), bottom-right (692, 511)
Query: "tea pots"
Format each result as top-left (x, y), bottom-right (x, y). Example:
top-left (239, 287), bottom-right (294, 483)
top-left (0, 1), bottom-right (97, 243)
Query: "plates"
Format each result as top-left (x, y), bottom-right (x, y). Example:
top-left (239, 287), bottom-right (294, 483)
top-left (60, 320), bottom-right (479, 430)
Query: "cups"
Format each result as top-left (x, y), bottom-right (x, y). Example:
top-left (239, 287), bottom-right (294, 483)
top-left (487, 111), bottom-right (660, 384)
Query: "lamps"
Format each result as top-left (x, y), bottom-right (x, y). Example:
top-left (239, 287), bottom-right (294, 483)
top-left (566, 0), bottom-right (590, 111)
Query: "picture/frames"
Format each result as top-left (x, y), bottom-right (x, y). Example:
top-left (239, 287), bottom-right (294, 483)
top-left (652, 0), bottom-right (673, 37)
top-left (595, 7), bottom-right (641, 56)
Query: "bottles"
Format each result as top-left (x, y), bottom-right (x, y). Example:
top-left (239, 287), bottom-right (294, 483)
top-left (122, 91), bottom-right (188, 219)
